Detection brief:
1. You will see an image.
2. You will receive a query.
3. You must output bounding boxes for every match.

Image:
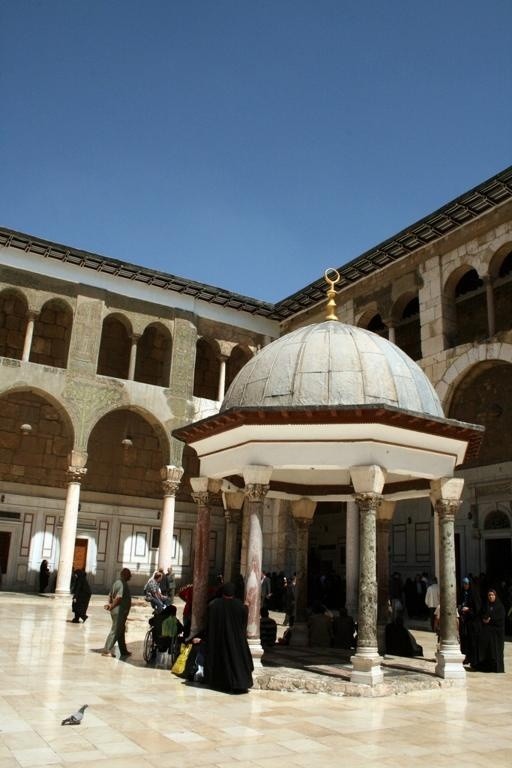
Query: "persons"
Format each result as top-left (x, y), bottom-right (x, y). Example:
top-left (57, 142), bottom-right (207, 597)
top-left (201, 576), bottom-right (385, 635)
top-left (383, 571), bottom-right (511, 673)
top-left (261, 570), bottom-right (357, 648)
top-left (101, 568), bottom-right (132, 659)
top-left (39, 560), bottom-right (50, 593)
top-left (144, 567), bottom-right (255, 693)
top-left (71, 569), bottom-right (93, 623)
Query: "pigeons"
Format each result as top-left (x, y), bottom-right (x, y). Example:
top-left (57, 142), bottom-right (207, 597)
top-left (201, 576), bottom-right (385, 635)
top-left (62, 704), bottom-right (88, 725)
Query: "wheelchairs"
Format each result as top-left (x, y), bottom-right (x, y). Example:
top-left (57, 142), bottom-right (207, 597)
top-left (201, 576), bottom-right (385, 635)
top-left (143, 626), bottom-right (181, 669)
